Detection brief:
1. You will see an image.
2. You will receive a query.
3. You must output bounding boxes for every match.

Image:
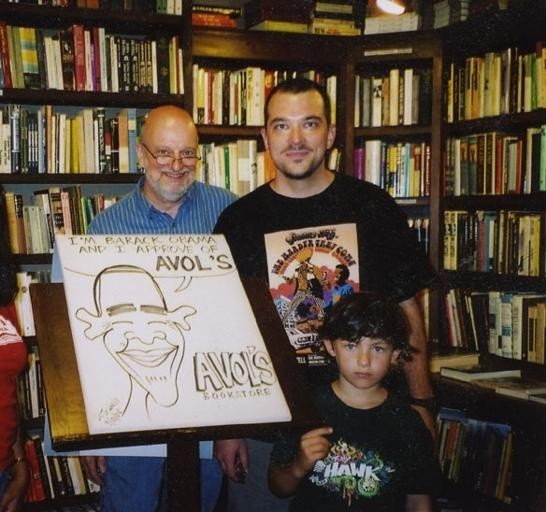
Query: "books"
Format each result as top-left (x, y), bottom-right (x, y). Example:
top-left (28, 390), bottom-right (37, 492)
top-left (20, 435), bottom-right (101, 500)
top-left (0, 24), bottom-right (185, 95)
top-left (441, 41), bottom-right (545, 363)
top-left (436, 405), bottom-right (514, 502)
top-left (0, 104), bottom-right (144, 175)
top-left (14, 353), bottom-right (46, 418)
top-left (429, 342), bottom-right (545, 403)
top-left (1, 185), bottom-right (120, 254)
top-left (13, 270), bottom-right (52, 336)
top-left (353, 68), bottom-right (430, 253)
top-left (194, 63), bottom-right (342, 199)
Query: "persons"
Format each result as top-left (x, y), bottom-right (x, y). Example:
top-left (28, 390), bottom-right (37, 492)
top-left (84, 104), bottom-right (239, 512)
top-left (269, 294), bottom-right (446, 512)
top-left (212, 78), bottom-right (440, 512)
top-left (0, 263), bottom-right (30, 512)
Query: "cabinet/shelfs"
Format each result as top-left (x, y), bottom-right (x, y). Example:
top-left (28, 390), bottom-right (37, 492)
top-left (0, 0), bottom-right (545, 512)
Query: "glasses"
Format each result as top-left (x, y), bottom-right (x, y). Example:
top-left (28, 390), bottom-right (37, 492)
top-left (141, 143), bottom-right (203, 167)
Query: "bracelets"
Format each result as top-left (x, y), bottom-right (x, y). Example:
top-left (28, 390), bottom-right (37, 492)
top-left (15, 457), bottom-right (27, 465)
